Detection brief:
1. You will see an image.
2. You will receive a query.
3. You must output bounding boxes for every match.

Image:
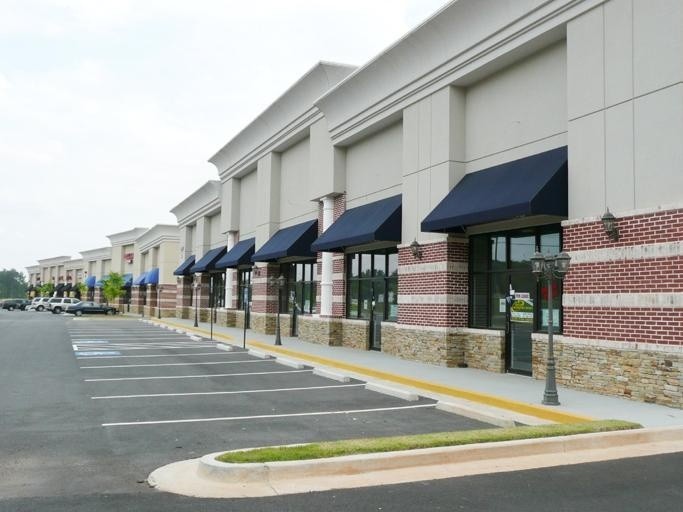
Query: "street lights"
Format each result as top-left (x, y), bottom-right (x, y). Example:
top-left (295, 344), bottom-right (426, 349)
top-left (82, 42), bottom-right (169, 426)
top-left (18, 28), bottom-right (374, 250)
top-left (191, 283), bottom-right (200, 327)
top-left (268, 276), bottom-right (287, 345)
top-left (532, 253), bottom-right (570, 406)
top-left (156, 287), bottom-right (164, 318)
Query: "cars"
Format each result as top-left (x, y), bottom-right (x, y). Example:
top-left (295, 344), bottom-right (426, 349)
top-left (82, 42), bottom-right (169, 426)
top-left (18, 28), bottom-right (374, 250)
top-left (2, 297), bottom-right (116, 316)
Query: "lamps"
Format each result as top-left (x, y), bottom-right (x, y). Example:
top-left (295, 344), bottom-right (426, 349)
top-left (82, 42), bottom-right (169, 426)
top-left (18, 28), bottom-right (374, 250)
top-left (409, 237), bottom-right (420, 257)
top-left (252, 266), bottom-right (260, 277)
top-left (600, 204), bottom-right (618, 240)
